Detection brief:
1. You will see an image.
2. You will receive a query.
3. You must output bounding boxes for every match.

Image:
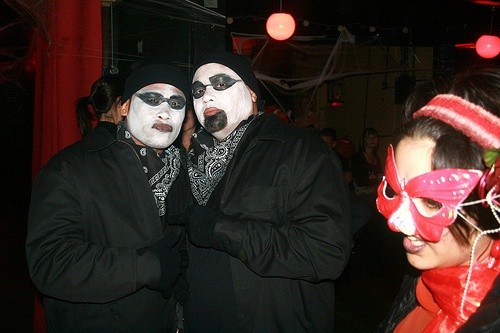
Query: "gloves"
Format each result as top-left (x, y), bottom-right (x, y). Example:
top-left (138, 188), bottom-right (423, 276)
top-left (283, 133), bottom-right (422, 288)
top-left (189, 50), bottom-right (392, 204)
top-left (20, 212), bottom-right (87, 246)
top-left (150, 225), bottom-right (184, 291)
top-left (186, 208), bottom-right (216, 247)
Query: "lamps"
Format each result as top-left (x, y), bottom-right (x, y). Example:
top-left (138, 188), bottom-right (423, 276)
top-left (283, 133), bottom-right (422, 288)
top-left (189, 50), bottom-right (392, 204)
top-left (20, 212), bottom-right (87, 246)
top-left (266, 0), bottom-right (296, 41)
top-left (475, 5), bottom-right (500, 58)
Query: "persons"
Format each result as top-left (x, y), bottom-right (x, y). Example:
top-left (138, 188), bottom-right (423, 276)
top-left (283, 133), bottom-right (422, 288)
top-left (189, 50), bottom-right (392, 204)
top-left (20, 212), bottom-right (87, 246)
top-left (75, 75), bottom-right (127, 138)
top-left (24, 62), bottom-right (192, 333)
top-left (167, 49), bottom-right (500, 333)
